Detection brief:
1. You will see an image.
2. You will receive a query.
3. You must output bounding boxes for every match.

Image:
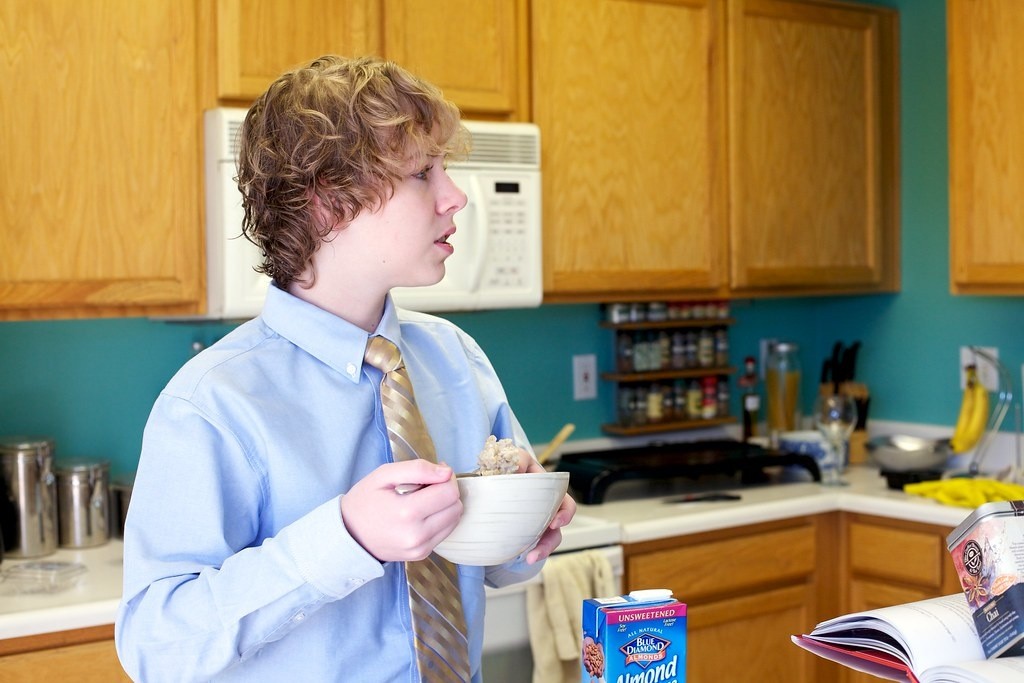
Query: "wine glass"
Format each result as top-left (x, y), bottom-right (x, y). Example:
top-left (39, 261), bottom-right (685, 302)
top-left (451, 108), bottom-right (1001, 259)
top-left (814, 396), bottom-right (858, 489)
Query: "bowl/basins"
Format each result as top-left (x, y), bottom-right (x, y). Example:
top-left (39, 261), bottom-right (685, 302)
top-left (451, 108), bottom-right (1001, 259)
top-left (777, 432), bottom-right (849, 478)
top-left (433, 472), bottom-right (569, 566)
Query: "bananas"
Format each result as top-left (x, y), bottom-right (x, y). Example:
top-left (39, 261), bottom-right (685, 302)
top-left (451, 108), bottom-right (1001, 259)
top-left (948, 364), bottom-right (988, 453)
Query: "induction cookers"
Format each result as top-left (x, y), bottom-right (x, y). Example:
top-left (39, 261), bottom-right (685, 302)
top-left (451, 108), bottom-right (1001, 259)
top-left (549, 438), bottom-right (821, 509)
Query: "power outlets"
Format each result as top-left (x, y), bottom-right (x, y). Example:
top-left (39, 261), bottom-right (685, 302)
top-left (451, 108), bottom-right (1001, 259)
top-left (959, 344), bottom-right (999, 391)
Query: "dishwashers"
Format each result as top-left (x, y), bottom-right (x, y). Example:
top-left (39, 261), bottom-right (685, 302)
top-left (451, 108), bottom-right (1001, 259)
top-left (477, 547), bottom-right (627, 683)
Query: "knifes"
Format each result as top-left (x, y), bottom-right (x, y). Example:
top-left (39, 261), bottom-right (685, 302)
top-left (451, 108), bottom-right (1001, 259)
top-left (820, 339), bottom-right (862, 386)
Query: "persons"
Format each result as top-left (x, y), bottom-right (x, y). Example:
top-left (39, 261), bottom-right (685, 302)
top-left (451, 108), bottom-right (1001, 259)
top-left (114, 56), bottom-right (576, 682)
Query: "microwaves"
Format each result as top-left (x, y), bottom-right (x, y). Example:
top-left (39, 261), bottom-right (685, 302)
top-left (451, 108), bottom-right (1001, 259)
top-left (203, 106), bottom-right (544, 319)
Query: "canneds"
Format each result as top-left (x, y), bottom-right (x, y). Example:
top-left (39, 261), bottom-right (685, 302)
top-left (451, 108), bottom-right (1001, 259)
top-left (603, 303), bottom-right (730, 423)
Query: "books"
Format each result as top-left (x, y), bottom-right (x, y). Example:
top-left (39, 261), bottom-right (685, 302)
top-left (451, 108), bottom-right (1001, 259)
top-left (791, 591), bottom-right (1024, 683)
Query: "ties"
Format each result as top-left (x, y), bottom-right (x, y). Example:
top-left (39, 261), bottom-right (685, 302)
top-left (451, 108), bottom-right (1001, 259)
top-left (363, 336), bottom-right (469, 683)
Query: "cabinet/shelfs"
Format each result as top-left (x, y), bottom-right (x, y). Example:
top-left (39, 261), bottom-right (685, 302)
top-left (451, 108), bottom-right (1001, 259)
top-left (947, 1), bottom-right (1023, 297)
top-left (2, 0), bottom-right (900, 318)
top-left (1, 511), bottom-right (967, 683)
top-left (600, 314), bottom-right (739, 433)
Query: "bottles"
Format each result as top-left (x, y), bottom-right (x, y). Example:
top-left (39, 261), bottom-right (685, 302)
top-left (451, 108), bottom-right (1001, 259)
top-left (609, 301), bottom-right (732, 320)
top-left (619, 329), bottom-right (728, 373)
top-left (615, 377), bottom-right (730, 427)
top-left (57, 458), bottom-right (110, 548)
top-left (0, 437), bottom-right (57, 558)
top-left (766, 343), bottom-right (802, 432)
